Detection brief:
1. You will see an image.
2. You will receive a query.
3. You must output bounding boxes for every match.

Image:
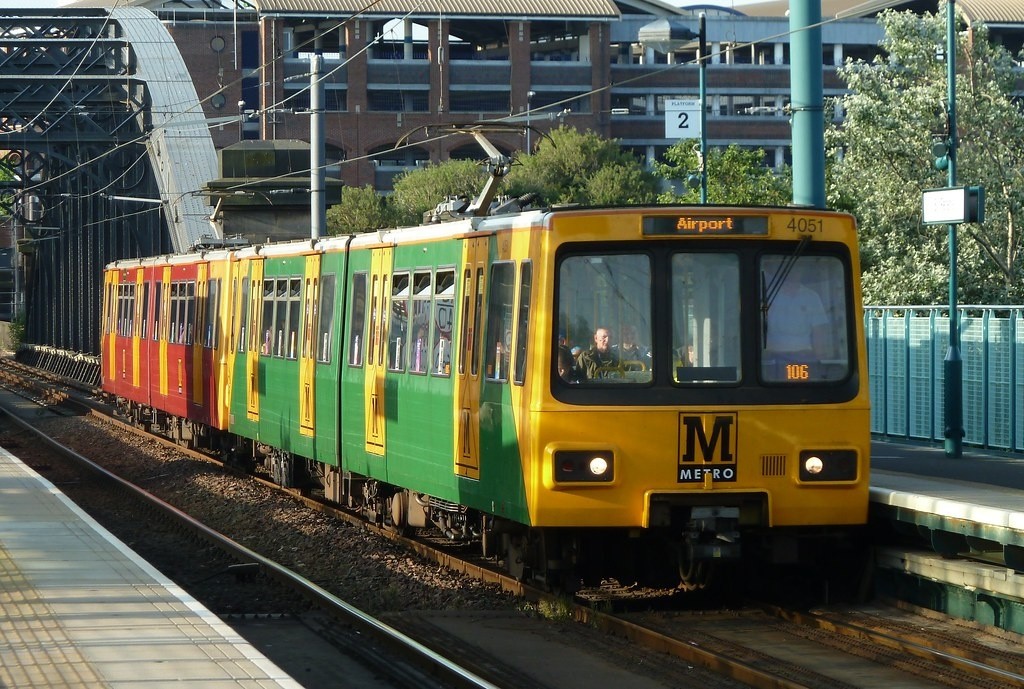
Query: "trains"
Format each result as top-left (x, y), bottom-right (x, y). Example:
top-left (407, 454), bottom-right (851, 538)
top-left (98, 120), bottom-right (872, 598)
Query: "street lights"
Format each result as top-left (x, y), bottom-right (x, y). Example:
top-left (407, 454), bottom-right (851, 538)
top-left (638, 12), bottom-right (707, 204)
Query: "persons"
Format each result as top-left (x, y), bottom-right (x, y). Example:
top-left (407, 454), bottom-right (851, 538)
top-left (762, 261), bottom-right (832, 380)
top-left (557, 323), bottom-right (649, 384)
top-left (400, 325), bottom-right (452, 375)
